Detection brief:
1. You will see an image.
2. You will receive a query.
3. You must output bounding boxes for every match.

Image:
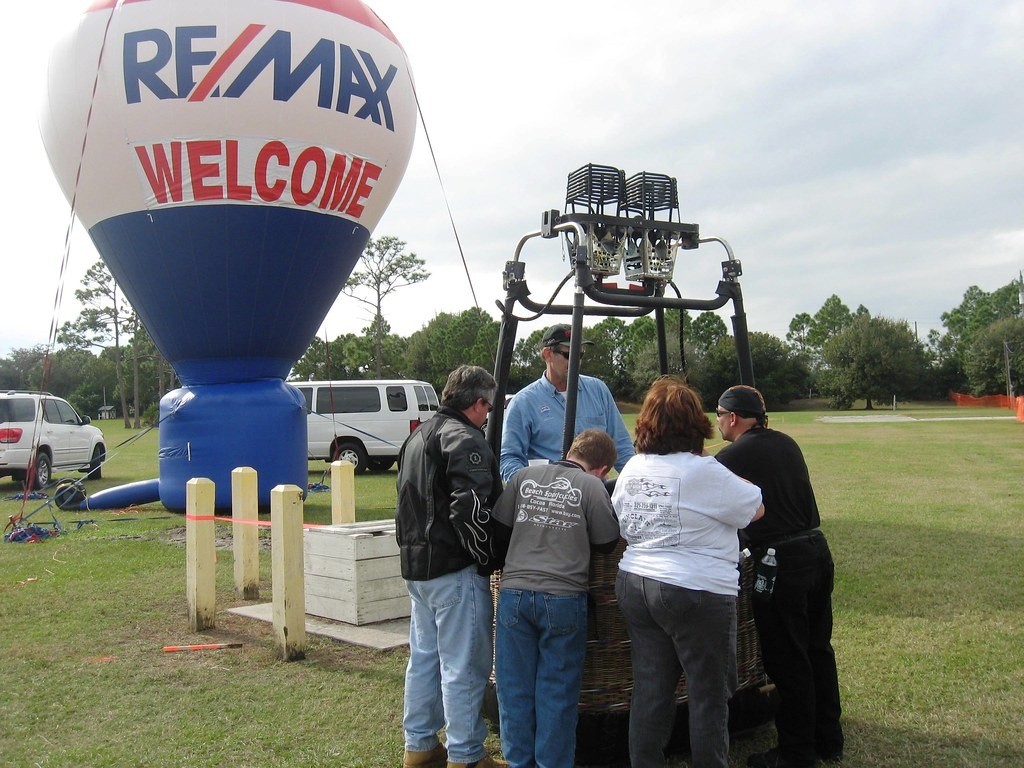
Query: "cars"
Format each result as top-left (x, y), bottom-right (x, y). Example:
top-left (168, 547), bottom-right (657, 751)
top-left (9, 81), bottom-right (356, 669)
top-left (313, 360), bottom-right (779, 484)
top-left (479, 394), bottom-right (514, 435)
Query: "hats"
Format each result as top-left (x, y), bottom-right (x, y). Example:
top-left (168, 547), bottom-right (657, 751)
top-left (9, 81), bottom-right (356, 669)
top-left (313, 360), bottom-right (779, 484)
top-left (541, 323), bottom-right (595, 348)
top-left (719, 384), bottom-right (766, 419)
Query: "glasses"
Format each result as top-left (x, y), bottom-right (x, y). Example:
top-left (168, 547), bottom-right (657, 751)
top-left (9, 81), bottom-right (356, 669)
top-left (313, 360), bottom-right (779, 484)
top-left (478, 396), bottom-right (494, 412)
top-left (716, 408), bottom-right (729, 417)
top-left (553, 350), bottom-right (585, 360)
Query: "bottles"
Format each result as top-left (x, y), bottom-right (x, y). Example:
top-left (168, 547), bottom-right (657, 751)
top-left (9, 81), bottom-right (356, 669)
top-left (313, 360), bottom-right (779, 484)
top-left (753, 549), bottom-right (778, 594)
top-left (738, 548), bottom-right (751, 565)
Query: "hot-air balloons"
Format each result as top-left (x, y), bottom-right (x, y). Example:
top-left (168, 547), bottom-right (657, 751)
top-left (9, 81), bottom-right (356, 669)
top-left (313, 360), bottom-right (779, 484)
top-left (40, 0), bottom-right (418, 515)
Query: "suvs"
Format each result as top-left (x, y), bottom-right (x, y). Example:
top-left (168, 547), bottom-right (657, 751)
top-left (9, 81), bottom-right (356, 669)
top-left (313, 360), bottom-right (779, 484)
top-left (0, 390), bottom-right (106, 493)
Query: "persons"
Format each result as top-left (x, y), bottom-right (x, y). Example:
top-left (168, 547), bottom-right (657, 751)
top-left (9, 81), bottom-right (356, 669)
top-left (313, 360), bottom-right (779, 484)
top-left (713, 386), bottom-right (841, 768)
top-left (610, 375), bottom-right (766, 768)
top-left (395, 364), bottom-right (501, 768)
top-left (498, 323), bottom-right (637, 483)
top-left (488, 430), bottom-right (620, 768)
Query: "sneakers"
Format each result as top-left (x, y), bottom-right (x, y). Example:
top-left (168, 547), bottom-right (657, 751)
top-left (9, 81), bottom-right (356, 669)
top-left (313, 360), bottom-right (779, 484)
top-left (402, 742), bottom-right (448, 768)
top-left (445, 753), bottom-right (509, 768)
top-left (748, 745), bottom-right (816, 768)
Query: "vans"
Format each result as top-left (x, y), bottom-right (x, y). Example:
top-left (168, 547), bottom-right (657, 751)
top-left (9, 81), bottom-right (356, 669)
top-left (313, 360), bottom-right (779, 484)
top-left (285, 380), bottom-right (439, 476)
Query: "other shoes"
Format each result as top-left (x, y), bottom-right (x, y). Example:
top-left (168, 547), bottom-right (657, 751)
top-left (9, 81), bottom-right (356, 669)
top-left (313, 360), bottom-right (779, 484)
top-left (816, 750), bottom-right (843, 760)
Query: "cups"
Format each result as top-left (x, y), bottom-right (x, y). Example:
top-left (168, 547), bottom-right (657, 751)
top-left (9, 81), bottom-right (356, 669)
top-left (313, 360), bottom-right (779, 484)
top-left (528, 459), bottom-right (550, 468)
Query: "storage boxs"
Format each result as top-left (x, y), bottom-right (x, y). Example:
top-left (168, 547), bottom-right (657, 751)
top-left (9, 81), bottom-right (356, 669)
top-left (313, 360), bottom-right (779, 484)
top-left (302, 518), bottom-right (411, 626)
